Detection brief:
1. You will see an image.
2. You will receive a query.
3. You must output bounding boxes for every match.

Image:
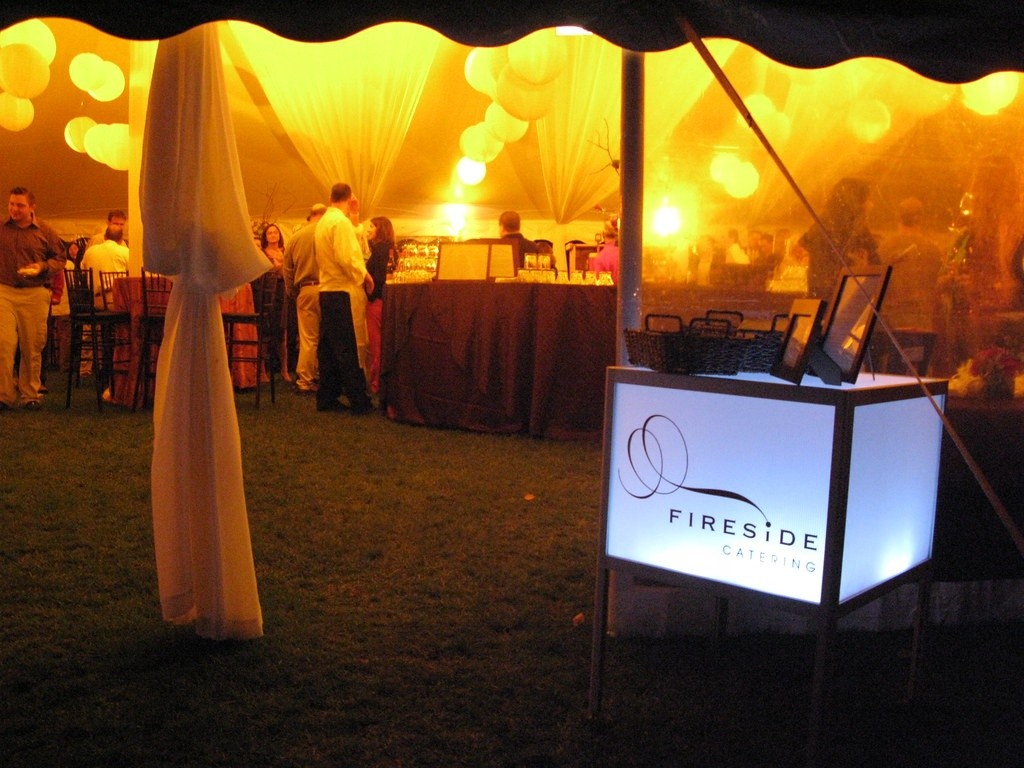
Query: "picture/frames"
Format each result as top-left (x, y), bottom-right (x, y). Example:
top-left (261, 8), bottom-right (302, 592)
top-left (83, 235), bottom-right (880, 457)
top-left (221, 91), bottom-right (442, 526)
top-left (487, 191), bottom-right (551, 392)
top-left (808, 265), bottom-right (893, 384)
top-left (769, 298), bottom-right (843, 387)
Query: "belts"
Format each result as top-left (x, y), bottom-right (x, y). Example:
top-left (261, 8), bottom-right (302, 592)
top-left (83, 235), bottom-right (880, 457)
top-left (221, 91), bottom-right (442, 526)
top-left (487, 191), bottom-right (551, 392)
top-left (301, 282), bottom-right (320, 287)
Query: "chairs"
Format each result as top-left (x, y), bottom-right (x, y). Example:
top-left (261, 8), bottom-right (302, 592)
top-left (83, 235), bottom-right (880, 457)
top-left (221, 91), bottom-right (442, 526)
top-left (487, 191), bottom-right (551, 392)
top-left (221, 271), bottom-right (281, 409)
top-left (64, 267), bottom-right (129, 412)
top-left (133, 267), bottom-right (173, 414)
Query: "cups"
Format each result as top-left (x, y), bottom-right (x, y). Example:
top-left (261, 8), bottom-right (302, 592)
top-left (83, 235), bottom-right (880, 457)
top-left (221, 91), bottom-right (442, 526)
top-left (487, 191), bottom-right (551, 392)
top-left (396, 242), bottom-right (438, 284)
top-left (538, 254), bottom-right (550, 269)
top-left (524, 253), bottom-right (536, 269)
top-left (569, 271), bottom-right (583, 284)
top-left (598, 271), bottom-right (613, 285)
top-left (543, 270), bottom-right (555, 282)
top-left (556, 271), bottom-right (569, 284)
top-left (530, 270), bottom-right (542, 282)
top-left (518, 270), bottom-right (530, 282)
top-left (584, 271), bottom-right (597, 285)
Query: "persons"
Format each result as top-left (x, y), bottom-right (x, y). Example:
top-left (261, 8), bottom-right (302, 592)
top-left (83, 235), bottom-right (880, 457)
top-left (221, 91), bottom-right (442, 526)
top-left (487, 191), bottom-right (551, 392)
top-left (284, 204), bottom-right (327, 390)
top-left (498, 211), bottom-right (541, 276)
top-left (0, 188), bottom-right (66, 410)
top-left (591, 217), bottom-right (618, 285)
top-left (799, 177), bottom-right (880, 301)
top-left (12, 210), bottom-right (129, 395)
top-left (315, 184), bottom-right (374, 414)
top-left (679, 228), bottom-right (773, 286)
top-left (878, 197), bottom-right (941, 332)
top-left (363, 218), bottom-right (397, 391)
top-left (259, 224), bottom-right (290, 383)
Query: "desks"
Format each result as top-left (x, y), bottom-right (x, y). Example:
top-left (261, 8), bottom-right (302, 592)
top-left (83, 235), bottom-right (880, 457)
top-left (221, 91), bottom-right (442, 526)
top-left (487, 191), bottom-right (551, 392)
top-left (110, 274), bottom-right (270, 399)
top-left (379, 283), bottom-right (616, 439)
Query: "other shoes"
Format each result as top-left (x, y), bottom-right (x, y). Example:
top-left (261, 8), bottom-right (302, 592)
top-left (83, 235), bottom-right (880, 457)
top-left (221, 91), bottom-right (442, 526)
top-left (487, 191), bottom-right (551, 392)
top-left (27, 401), bottom-right (40, 411)
top-left (281, 371), bottom-right (293, 383)
top-left (318, 399), bottom-right (348, 412)
top-left (260, 372), bottom-right (269, 383)
top-left (362, 404), bottom-right (377, 415)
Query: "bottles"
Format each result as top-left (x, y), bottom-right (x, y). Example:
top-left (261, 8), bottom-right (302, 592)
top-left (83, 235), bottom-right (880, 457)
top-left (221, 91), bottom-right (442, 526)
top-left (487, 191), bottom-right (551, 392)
top-left (385, 249), bottom-right (397, 281)
